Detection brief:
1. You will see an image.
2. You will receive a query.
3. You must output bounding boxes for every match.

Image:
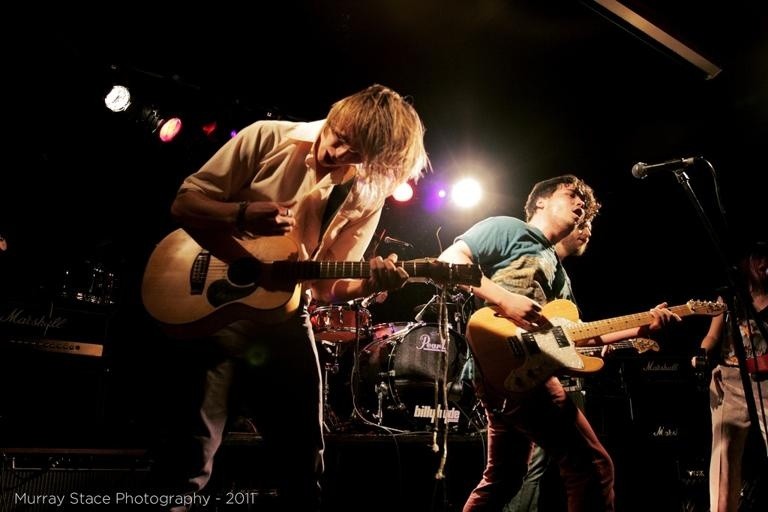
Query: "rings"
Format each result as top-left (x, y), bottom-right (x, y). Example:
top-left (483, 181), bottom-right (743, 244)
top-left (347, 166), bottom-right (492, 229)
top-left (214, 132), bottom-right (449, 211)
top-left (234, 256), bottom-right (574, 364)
top-left (286, 208), bottom-right (292, 215)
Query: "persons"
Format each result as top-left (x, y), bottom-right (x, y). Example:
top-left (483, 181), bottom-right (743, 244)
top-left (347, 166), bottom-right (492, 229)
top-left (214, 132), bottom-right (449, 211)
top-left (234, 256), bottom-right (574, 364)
top-left (691, 242), bottom-right (768, 512)
top-left (171, 84), bottom-right (426, 511)
top-left (437, 175), bottom-right (682, 512)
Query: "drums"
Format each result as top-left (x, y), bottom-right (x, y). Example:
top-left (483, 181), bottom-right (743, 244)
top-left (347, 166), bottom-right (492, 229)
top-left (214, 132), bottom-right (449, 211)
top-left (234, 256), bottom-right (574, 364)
top-left (351, 323), bottom-right (477, 434)
top-left (370, 322), bottom-right (408, 339)
top-left (310, 305), bottom-right (371, 342)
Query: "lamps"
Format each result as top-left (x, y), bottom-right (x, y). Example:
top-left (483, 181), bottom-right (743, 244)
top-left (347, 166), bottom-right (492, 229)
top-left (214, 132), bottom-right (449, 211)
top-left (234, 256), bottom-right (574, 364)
top-left (141, 108), bottom-right (182, 142)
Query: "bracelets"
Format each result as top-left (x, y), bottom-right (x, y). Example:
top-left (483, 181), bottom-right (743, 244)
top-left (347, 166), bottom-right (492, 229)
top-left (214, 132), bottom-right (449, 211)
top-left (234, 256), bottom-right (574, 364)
top-left (237, 200), bottom-right (255, 239)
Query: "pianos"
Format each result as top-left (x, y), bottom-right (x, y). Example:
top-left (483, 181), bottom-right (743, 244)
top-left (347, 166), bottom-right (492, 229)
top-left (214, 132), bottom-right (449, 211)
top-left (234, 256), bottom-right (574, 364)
top-left (6, 338), bottom-right (102, 360)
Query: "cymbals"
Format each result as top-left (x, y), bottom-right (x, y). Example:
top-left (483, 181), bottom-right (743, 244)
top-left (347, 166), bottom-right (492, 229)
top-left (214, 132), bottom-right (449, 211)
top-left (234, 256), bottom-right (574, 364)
top-left (414, 302), bottom-right (455, 311)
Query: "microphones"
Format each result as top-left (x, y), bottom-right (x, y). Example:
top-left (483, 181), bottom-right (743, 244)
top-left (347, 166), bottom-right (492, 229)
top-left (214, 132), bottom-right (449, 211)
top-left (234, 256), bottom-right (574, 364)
top-left (632, 155), bottom-right (704, 179)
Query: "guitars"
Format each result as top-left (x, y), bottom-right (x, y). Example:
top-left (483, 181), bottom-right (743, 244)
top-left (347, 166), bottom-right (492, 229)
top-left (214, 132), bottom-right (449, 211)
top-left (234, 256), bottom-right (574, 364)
top-left (465, 298), bottom-right (727, 415)
top-left (676, 348), bottom-right (711, 512)
top-left (140, 229), bottom-right (481, 335)
